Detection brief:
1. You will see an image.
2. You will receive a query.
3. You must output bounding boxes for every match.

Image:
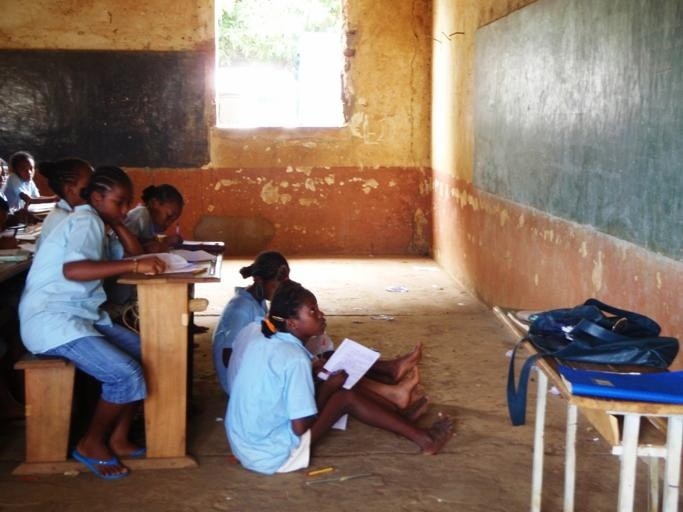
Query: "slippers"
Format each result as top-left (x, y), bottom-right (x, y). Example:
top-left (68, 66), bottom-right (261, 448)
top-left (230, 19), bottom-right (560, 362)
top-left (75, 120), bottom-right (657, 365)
top-left (69, 443), bottom-right (129, 479)
top-left (121, 447), bottom-right (147, 459)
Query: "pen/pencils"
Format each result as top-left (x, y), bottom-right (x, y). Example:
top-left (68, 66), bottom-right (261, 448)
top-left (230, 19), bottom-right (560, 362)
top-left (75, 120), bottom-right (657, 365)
top-left (319, 367), bottom-right (332, 377)
top-left (176, 224), bottom-right (179, 234)
top-left (13, 228), bottom-right (16, 236)
top-left (309, 467), bottom-right (333, 475)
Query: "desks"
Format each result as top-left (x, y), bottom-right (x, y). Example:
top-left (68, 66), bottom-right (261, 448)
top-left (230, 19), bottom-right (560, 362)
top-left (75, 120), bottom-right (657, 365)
top-left (0, 217), bottom-right (48, 281)
top-left (115, 238), bottom-right (225, 471)
top-left (493, 308), bottom-right (683, 512)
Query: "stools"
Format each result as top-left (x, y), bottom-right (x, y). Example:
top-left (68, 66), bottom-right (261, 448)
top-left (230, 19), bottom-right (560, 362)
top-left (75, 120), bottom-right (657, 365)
top-left (11, 356), bottom-right (83, 476)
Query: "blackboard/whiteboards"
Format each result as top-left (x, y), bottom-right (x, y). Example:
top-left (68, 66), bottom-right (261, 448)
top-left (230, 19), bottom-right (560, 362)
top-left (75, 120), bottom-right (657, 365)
top-left (471, 0), bottom-right (682, 264)
top-left (0, 48), bottom-right (212, 166)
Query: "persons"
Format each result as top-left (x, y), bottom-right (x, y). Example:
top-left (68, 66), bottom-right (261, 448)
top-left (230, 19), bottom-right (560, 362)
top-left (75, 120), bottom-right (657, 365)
top-left (37, 161), bottom-right (130, 327)
top-left (19, 166), bottom-right (167, 479)
top-left (127, 183), bottom-right (182, 252)
top-left (1, 151), bottom-right (60, 249)
top-left (224, 281), bottom-right (454, 474)
top-left (212, 251), bottom-right (424, 395)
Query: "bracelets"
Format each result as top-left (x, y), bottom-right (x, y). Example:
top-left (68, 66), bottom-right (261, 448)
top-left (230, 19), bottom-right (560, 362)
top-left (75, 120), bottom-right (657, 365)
top-left (133, 257), bottom-right (137, 274)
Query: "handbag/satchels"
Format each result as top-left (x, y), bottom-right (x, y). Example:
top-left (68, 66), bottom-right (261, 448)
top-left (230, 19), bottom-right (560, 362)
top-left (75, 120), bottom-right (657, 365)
top-left (506, 298), bottom-right (679, 428)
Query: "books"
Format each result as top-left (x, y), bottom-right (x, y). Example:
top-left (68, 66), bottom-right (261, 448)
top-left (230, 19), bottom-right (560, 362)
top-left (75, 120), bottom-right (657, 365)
top-left (556, 365), bottom-right (682, 405)
top-left (124, 240), bottom-right (225, 274)
